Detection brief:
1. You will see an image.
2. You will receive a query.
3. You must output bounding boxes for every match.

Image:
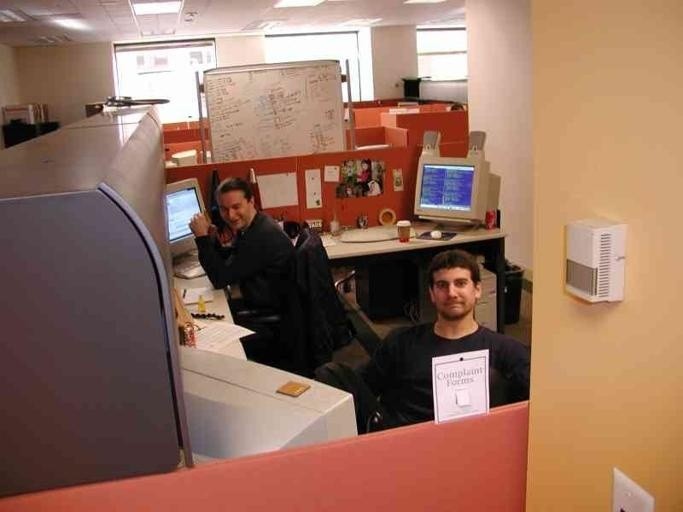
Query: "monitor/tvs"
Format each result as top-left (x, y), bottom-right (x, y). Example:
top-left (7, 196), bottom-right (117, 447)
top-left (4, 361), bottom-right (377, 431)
top-left (179, 345), bottom-right (359, 461)
top-left (414, 156), bottom-right (501, 233)
top-left (166, 149), bottom-right (197, 168)
top-left (167, 177), bottom-right (211, 258)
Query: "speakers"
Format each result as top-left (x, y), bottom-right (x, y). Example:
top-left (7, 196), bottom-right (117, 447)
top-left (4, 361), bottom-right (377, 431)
top-left (421, 131), bottom-right (441, 157)
top-left (466, 131), bottom-right (485, 161)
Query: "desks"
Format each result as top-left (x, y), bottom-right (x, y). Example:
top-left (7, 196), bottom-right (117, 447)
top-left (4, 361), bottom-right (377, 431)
top-left (171, 218), bottom-right (509, 472)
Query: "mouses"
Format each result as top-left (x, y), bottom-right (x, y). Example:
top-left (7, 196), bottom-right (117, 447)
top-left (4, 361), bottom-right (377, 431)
top-left (430, 230), bottom-right (442, 239)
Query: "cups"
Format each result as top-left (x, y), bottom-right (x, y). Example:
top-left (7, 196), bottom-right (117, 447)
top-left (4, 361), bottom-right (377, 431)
top-left (397, 221), bottom-right (412, 243)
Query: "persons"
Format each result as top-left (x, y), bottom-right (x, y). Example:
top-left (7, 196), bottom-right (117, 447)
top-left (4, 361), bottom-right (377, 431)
top-left (345, 159), bottom-right (381, 198)
top-left (189, 177), bottom-right (295, 359)
top-left (317, 251), bottom-right (530, 435)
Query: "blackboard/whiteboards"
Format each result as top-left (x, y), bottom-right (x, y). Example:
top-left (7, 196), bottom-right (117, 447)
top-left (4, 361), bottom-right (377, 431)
top-left (203, 60), bottom-right (347, 162)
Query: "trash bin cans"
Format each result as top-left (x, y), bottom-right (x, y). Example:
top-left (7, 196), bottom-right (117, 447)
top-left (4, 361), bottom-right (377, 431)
top-left (503, 261), bottom-right (524, 325)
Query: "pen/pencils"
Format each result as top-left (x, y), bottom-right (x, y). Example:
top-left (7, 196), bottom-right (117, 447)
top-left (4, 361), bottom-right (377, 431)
top-left (182, 289), bottom-right (186, 298)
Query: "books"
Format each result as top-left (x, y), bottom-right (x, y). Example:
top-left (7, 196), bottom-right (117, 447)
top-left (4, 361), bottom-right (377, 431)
top-left (180, 287), bottom-right (214, 304)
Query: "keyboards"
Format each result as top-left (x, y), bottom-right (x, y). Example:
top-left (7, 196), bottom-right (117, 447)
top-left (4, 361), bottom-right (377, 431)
top-left (341, 225), bottom-right (415, 243)
top-left (173, 256), bottom-right (207, 279)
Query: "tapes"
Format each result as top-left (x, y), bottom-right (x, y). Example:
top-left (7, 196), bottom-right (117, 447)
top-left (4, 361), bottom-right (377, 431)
top-left (379, 208), bottom-right (395, 225)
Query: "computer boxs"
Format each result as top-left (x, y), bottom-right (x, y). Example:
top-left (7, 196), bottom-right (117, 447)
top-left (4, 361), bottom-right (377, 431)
top-left (420, 255), bottom-right (498, 336)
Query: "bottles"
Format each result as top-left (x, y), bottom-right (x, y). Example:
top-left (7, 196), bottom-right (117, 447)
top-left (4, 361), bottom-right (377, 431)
top-left (198, 295), bottom-right (207, 313)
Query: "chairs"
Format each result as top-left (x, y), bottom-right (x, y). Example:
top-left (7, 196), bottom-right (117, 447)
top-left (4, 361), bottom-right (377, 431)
top-left (289, 227), bottom-right (357, 376)
